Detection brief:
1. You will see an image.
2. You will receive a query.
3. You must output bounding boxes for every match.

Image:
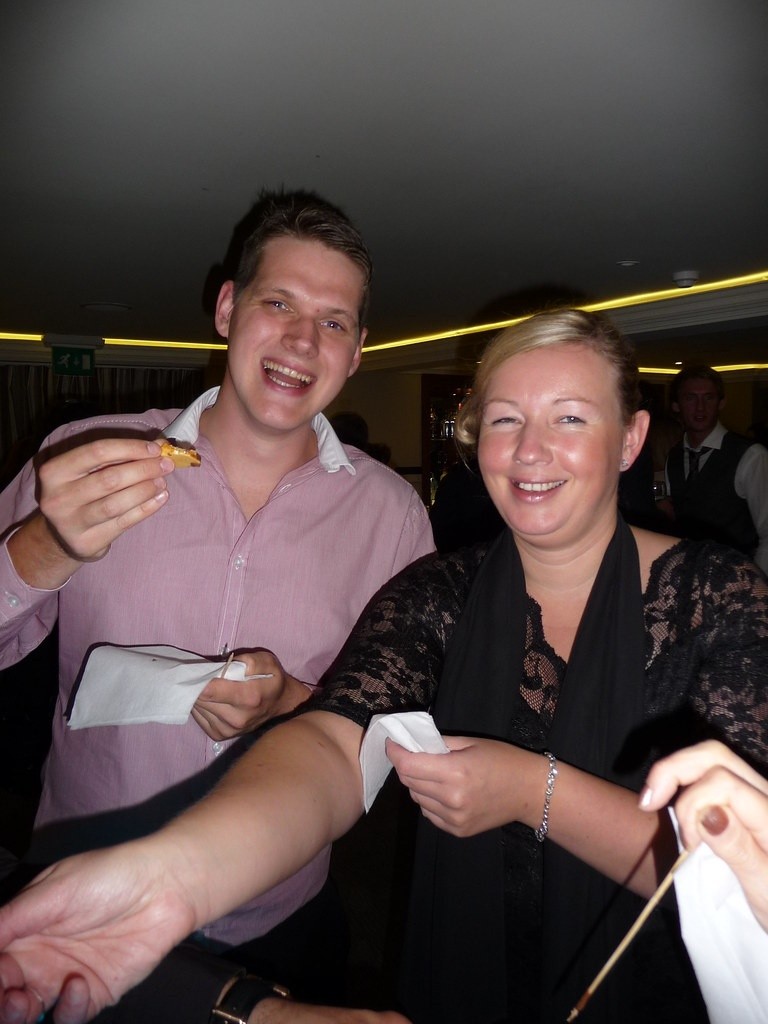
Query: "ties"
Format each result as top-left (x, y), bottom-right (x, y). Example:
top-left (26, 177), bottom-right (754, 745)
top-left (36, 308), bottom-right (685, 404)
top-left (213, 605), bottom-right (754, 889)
top-left (685, 446), bottom-right (713, 482)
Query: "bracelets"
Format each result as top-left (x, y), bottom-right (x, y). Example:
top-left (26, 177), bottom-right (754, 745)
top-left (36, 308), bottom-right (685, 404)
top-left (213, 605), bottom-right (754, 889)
top-left (211, 974), bottom-right (292, 1024)
top-left (534, 752), bottom-right (557, 843)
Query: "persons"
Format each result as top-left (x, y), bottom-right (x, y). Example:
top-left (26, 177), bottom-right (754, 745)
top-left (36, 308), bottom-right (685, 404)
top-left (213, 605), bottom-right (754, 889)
top-left (0, 186), bottom-right (437, 1024)
top-left (329, 411), bottom-right (503, 548)
top-left (0, 304), bottom-right (767, 1024)
top-left (618, 364), bottom-right (768, 577)
